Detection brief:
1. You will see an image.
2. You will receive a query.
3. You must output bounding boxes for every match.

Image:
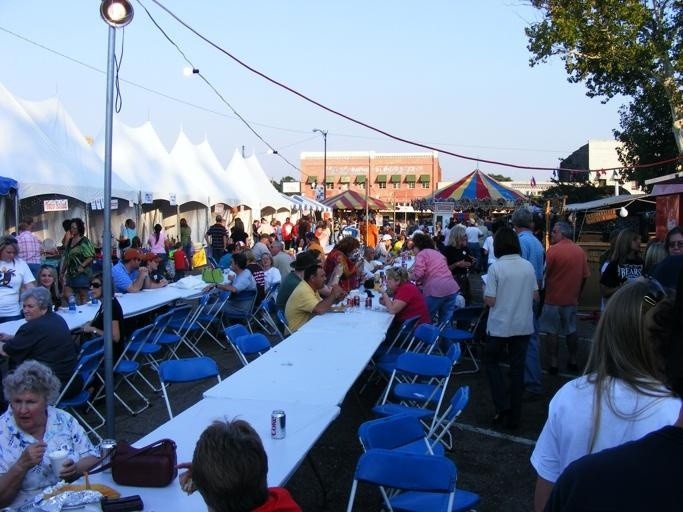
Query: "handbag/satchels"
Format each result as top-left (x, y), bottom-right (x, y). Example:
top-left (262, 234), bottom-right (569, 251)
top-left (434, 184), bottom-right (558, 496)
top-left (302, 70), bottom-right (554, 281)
top-left (112, 439), bottom-right (178, 487)
top-left (201, 268), bottom-right (224, 283)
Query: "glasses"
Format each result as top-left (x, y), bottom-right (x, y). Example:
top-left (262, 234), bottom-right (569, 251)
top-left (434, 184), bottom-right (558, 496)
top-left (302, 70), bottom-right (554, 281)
top-left (89, 282), bottom-right (102, 289)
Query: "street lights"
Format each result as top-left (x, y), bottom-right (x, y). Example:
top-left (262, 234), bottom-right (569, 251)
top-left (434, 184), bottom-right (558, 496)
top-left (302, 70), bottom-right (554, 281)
top-left (94, 0), bottom-right (143, 445)
top-left (611, 174), bottom-right (622, 197)
top-left (310, 125), bottom-right (329, 200)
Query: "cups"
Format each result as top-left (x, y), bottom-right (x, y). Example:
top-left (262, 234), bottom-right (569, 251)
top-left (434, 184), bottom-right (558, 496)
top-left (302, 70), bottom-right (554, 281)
top-left (48, 449), bottom-right (70, 477)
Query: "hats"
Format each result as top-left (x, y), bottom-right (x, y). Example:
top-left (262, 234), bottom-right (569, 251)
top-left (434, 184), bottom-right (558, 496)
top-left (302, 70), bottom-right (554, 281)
top-left (290, 252), bottom-right (320, 269)
top-left (125, 248), bottom-right (148, 260)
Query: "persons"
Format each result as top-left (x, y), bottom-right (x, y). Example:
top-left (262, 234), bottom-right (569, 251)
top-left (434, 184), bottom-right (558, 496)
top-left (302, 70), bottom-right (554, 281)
top-left (1, 271), bottom-right (124, 510)
top-left (372, 206), bottom-right (592, 423)
top-left (530, 223), bottom-right (682, 511)
top-left (174, 415), bottom-right (300, 511)
top-left (1, 210), bottom-right (379, 334)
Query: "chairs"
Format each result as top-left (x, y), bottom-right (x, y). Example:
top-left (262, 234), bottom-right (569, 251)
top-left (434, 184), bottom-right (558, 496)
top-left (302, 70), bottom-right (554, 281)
top-left (2, 258), bottom-right (287, 415)
top-left (345, 298), bottom-right (493, 511)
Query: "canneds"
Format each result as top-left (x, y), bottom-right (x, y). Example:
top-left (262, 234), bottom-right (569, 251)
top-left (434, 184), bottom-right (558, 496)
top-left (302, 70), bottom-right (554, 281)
top-left (354, 295), bottom-right (360, 309)
top-left (100, 439), bottom-right (117, 474)
top-left (271, 410), bottom-right (286, 440)
top-left (347, 298), bottom-right (352, 309)
top-left (365, 297), bottom-right (372, 310)
top-left (402, 263), bottom-right (407, 268)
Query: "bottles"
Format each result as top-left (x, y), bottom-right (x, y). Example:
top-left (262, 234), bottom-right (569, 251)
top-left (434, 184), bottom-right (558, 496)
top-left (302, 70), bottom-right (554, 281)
top-left (67, 289), bottom-right (97, 314)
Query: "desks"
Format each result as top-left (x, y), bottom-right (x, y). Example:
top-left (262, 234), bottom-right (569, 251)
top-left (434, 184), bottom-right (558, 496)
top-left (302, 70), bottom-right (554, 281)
top-left (202, 330), bottom-right (388, 495)
top-left (39, 398), bottom-right (340, 512)
top-left (309, 246), bottom-right (423, 313)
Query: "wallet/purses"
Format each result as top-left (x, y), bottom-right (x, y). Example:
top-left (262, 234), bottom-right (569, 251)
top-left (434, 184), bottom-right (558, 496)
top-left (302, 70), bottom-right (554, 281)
top-left (101, 495), bottom-right (143, 512)
